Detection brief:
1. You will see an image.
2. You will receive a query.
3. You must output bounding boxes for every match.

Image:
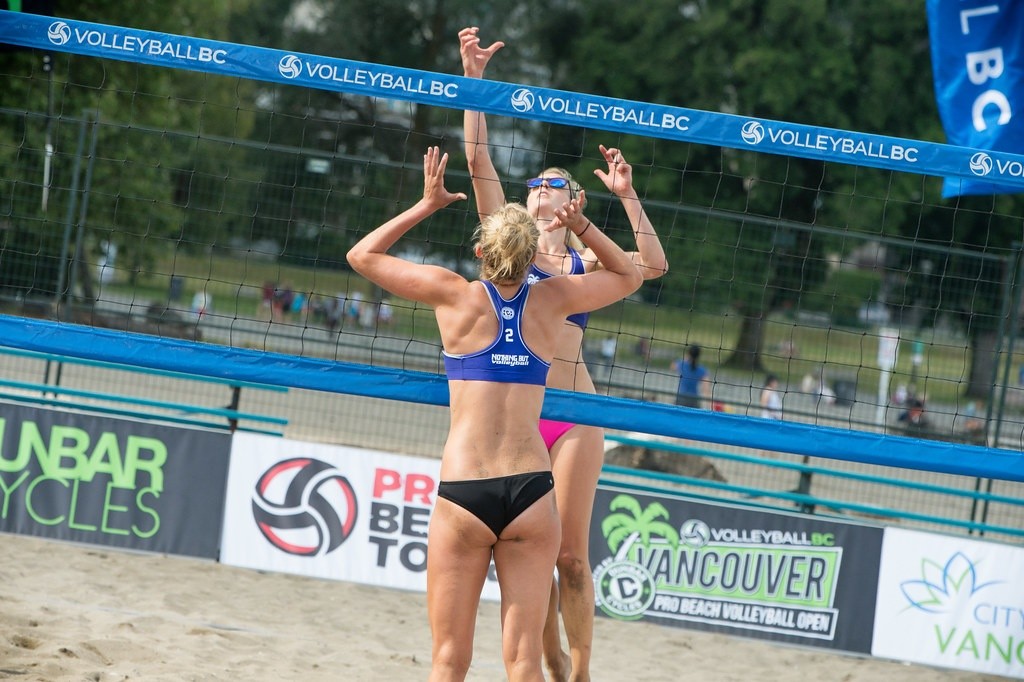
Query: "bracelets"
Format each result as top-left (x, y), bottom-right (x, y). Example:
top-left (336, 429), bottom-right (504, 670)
top-left (576, 221), bottom-right (590, 236)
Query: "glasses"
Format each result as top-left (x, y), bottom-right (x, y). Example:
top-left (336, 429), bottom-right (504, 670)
top-left (526, 177), bottom-right (573, 201)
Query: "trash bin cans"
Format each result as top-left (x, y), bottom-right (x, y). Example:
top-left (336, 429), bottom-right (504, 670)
top-left (170, 278), bottom-right (183, 299)
top-left (834, 379), bottom-right (858, 406)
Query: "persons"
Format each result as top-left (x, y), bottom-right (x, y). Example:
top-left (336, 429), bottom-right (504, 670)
top-left (345, 147), bottom-right (645, 682)
top-left (92, 249), bottom-right (986, 444)
top-left (459, 27), bottom-right (668, 682)
